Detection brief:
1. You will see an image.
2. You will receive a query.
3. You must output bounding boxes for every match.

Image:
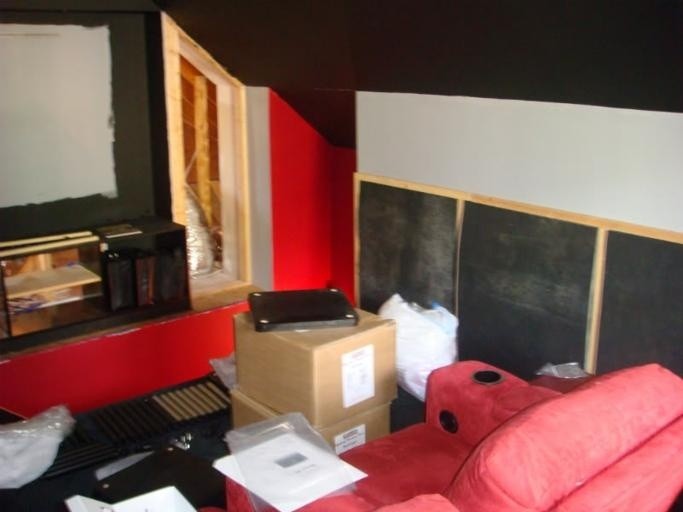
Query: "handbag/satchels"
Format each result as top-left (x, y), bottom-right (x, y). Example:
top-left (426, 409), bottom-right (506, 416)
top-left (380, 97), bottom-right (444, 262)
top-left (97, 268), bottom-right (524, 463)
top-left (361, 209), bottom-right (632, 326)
top-left (377, 293), bottom-right (459, 403)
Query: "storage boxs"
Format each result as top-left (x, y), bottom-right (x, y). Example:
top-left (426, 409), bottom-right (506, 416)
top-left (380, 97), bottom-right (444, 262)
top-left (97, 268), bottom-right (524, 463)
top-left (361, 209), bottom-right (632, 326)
top-left (226, 389), bottom-right (392, 458)
top-left (234, 305), bottom-right (399, 430)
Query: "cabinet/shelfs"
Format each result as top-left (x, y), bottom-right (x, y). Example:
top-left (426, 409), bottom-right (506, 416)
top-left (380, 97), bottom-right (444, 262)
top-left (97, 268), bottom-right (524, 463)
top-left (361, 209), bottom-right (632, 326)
top-left (0, 216), bottom-right (194, 357)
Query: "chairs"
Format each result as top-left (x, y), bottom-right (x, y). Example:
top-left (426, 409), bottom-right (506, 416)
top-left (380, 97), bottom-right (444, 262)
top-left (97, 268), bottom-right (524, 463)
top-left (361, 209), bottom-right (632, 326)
top-left (223, 360), bottom-right (683, 512)
